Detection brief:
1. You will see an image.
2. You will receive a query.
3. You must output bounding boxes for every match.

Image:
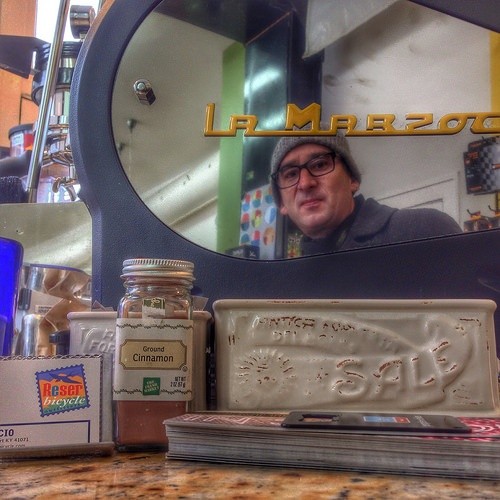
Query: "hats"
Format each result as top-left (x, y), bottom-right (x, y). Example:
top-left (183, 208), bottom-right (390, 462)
top-left (269, 120), bottom-right (361, 207)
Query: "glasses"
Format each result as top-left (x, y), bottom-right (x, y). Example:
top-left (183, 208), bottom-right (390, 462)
top-left (271, 152), bottom-right (336, 189)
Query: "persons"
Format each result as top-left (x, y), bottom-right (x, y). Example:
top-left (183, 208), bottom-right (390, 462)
top-left (268, 121), bottom-right (465, 258)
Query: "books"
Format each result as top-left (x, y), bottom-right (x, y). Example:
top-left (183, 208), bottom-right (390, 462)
top-left (162, 410), bottom-right (500, 481)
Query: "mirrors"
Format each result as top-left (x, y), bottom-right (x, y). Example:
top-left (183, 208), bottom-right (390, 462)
top-left (68, 0), bottom-right (500, 312)
